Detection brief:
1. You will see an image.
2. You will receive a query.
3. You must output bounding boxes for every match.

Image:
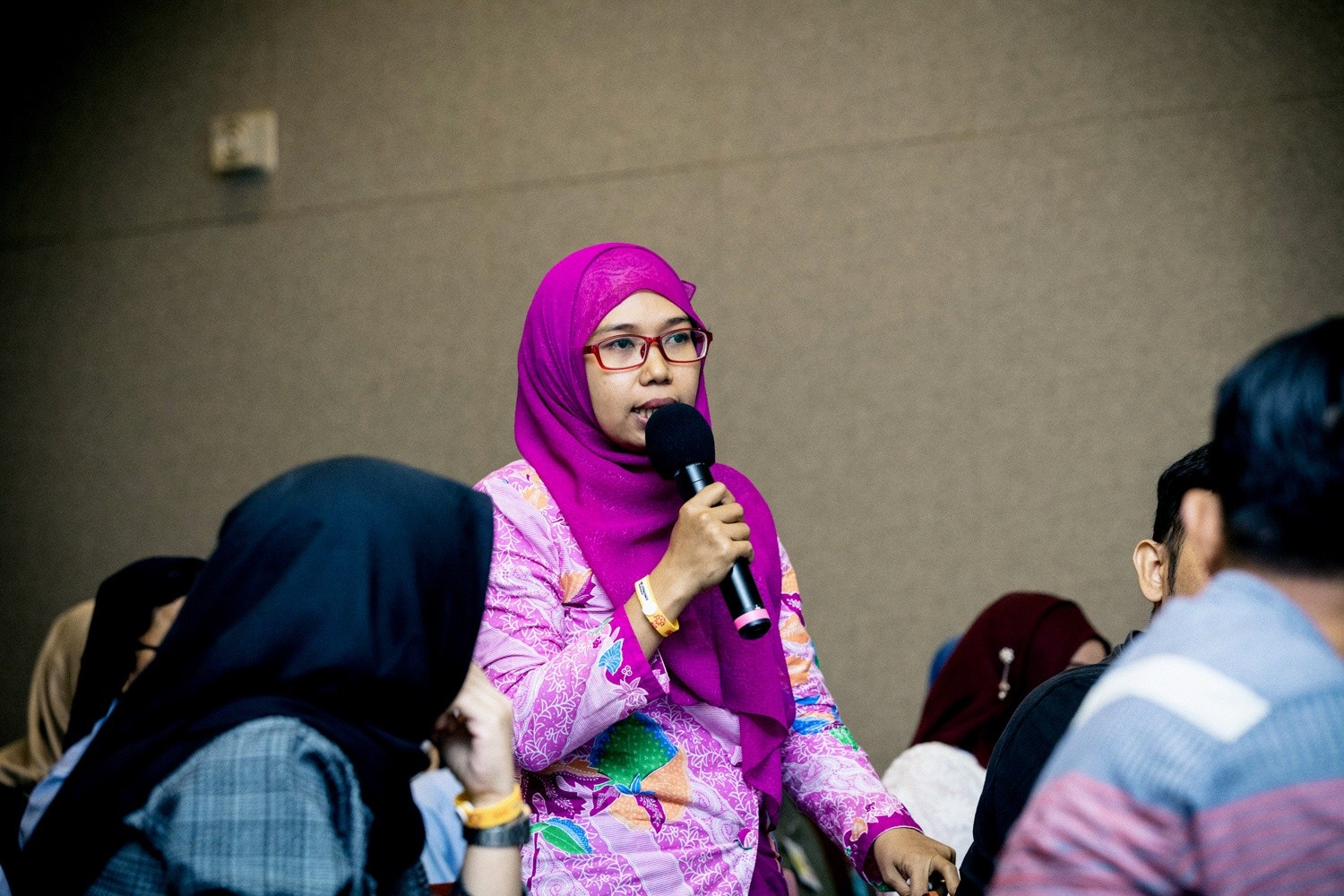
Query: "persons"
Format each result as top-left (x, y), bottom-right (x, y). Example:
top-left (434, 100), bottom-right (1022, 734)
top-left (469, 242), bottom-right (958, 896)
top-left (875, 316), bottom-right (1344, 896)
top-left (0, 453), bottom-right (542, 896)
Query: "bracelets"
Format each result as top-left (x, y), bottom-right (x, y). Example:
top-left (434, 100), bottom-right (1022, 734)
top-left (460, 813), bottom-right (533, 847)
top-left (635, 575), bottom-right (679, 638)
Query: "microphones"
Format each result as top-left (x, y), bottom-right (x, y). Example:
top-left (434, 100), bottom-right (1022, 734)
top-left (646, 403), bottom-right (772, 641)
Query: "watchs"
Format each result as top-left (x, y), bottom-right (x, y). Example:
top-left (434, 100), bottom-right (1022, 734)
top-left (455, 779), bottom-right (524, 829)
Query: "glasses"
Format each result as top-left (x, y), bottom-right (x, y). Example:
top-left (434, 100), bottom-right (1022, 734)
top-left (582, 327), bottom-right (713, 371)
